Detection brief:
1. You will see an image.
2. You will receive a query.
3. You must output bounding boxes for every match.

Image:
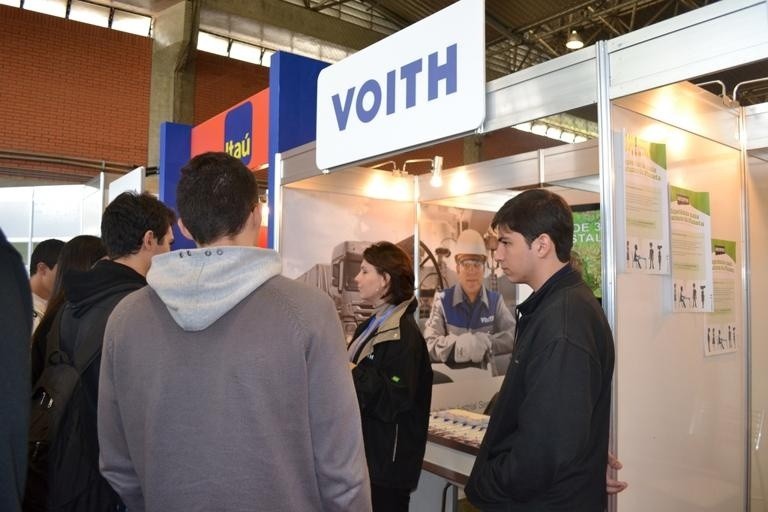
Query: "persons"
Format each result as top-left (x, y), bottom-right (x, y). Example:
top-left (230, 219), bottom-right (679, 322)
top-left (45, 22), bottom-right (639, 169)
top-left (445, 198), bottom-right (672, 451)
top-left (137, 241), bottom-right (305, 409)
top-left (464, 189), bottom-right (627, 511)
top-left (27, 239), bottom-right (68, 345)
top-left (99, 152), bottom-right (370, 512)
top-left (424, 231), bottom-right (516, 365)
top-left (27, 192), bottom-right (177, 511)
top-left (344, 241), bottom-right (432, 511)
top-left (0, 229), bottom-right (33, 511)
top-left (36, 237), bottom-right (106, 378)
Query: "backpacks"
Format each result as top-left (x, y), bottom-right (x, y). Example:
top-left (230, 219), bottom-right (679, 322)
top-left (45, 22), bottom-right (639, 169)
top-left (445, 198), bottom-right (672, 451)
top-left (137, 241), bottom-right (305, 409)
top-left (28, 297), bottom-right (104, 512)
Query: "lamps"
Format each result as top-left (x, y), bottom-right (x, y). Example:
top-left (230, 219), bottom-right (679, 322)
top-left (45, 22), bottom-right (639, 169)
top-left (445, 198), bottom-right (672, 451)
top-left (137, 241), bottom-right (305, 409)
top-left (402, 156), bottom-right (443, 187)
top-left (565, 25), bottom-right (585, 49)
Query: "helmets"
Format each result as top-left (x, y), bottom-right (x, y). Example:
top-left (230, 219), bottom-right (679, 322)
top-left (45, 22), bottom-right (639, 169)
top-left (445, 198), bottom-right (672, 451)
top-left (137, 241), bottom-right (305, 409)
top-left (452, 228), bottom-right (488, 260)
